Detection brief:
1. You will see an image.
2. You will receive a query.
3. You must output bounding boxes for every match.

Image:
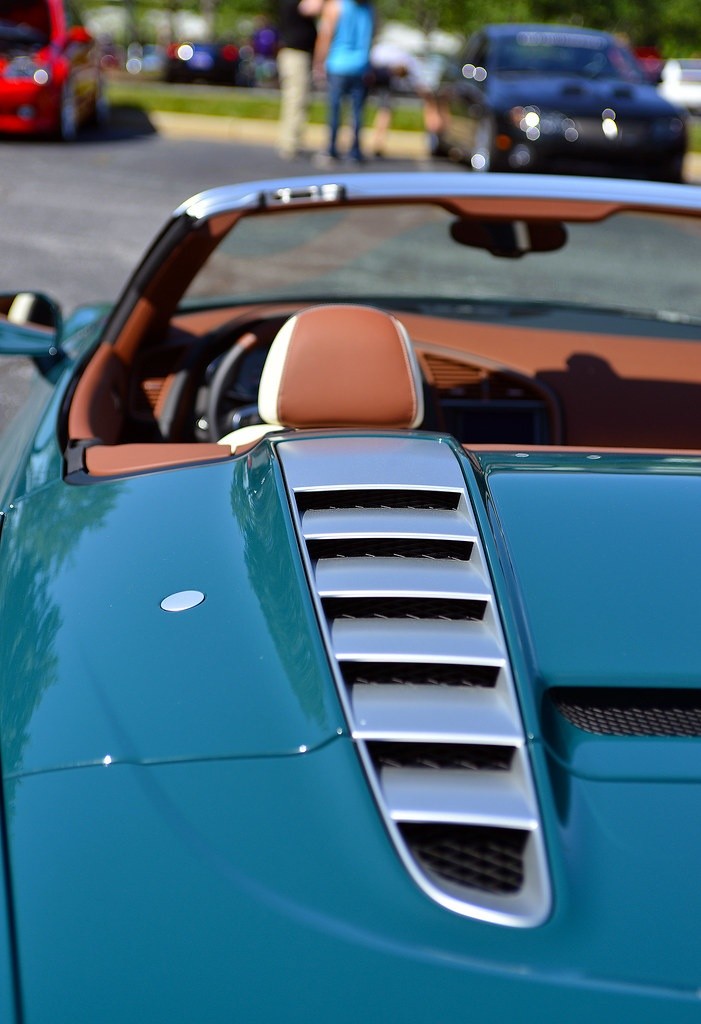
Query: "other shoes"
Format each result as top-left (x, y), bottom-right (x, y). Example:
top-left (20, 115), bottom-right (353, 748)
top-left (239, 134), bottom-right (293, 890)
top-left (311, 151), bottom-right (338, 170)
top-left (345, 154), bottom-right (367, 169)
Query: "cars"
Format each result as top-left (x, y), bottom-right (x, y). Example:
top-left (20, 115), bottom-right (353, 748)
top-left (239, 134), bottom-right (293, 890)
top-left (165, 40), bottom-right (251, 84)
top-left (429, 23), bottom-right (686, 179)
top-left (0, 0), bottom-right (102, 138)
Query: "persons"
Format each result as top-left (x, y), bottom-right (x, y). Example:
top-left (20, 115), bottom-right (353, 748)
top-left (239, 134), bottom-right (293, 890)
top-left (324, 0), bottom-right (376, 162)
top-left (273, 0), bottom-right (324, 160)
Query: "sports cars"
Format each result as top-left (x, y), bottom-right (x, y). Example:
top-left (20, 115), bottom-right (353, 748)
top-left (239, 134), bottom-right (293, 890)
top-left (0, 172), bottom-right (700, 1024)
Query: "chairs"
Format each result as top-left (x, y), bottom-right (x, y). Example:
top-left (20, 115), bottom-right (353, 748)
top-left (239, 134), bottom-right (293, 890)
top-left (216, 304), bottom-right (425, 454)
